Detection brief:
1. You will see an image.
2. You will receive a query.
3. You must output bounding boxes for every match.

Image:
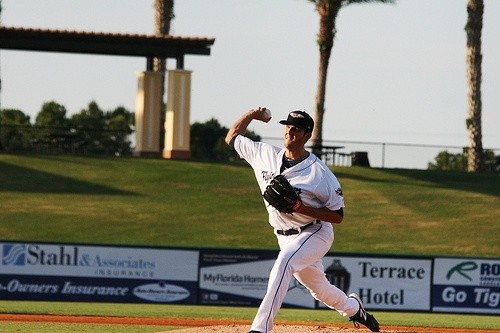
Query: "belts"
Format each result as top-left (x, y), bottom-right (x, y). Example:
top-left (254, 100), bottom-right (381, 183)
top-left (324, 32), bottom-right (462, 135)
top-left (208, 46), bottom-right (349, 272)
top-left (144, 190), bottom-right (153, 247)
top-left (277, 220), bottom-right (321, 235)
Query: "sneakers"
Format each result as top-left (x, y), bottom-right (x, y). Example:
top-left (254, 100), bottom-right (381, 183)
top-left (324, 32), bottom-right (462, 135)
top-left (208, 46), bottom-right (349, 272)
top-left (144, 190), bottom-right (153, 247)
top-left (349, 293), bottom-right (380, 332)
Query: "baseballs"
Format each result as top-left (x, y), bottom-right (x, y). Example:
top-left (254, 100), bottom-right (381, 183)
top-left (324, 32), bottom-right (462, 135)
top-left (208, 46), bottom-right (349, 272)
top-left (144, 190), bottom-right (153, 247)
top-left (264, 109), bottom-right (271, 119)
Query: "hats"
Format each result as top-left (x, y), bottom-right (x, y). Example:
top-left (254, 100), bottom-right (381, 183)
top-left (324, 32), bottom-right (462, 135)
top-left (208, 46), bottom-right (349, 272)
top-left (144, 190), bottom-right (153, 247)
top-left (279, 111), bottom-right (314, 133)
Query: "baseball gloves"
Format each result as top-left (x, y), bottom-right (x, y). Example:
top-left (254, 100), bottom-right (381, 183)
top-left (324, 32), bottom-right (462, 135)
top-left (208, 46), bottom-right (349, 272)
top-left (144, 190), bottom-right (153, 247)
top-left (261, 174), bottom-right (302, 216)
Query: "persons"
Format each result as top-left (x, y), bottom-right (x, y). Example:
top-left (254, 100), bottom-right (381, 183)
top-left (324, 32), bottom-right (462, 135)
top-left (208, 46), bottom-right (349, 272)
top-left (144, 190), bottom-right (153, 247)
top-left (224, 107), bottom-right (380, 333)
